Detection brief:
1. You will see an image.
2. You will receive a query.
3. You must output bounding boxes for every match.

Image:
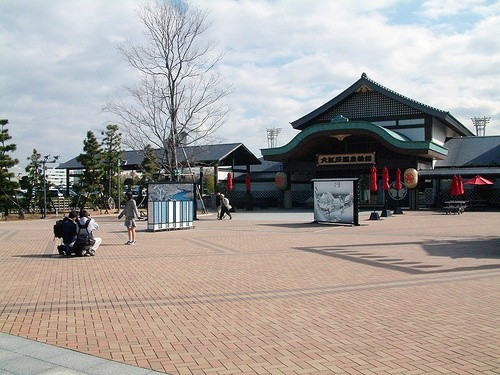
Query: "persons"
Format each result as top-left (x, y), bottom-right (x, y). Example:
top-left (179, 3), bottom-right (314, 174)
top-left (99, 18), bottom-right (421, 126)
top-left (117, 191), bottom-right (144, 245)
top-left (216, 192), bottom-right (226, 218)
top-left (218, 194), bottom-right (233, 220)
top-left (54, 209), bottom-right (102, 257)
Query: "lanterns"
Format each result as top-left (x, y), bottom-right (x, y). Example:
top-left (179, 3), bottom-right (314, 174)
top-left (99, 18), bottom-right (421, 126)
top-left (275, 171), bottom-right (287, 190)
top-left (404, 168), bottom-right (418, 188)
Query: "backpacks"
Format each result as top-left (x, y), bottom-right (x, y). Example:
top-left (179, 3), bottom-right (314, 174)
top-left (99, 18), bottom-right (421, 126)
top-left (75, 219), bottom-right (95, 248)
top-left (53, 218), bottom-right (67, 239)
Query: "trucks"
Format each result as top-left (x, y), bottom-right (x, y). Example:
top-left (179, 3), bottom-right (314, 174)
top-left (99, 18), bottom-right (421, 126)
top-left (123, 177), bottom-right (148, 205)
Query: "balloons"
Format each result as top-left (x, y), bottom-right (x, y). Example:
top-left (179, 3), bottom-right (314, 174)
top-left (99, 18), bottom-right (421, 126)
top-left (227, 172), bottom-right (233, 200)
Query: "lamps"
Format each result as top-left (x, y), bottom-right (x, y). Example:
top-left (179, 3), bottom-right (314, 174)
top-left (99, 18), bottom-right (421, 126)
top-left (328, 134), bottom-right (352, 141)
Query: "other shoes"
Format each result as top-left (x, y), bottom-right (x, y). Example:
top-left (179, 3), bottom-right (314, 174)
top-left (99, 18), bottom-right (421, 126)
top-left (80, 249), bottom-right (90, 257)
top-left (56, 245), bottom-right (64, 255)
top-left (90, 250), bottom-right (95, 256)
top-left (65, 248), bottom-right (71, 257)
top-left (124, 240), bottom-right (136, 245)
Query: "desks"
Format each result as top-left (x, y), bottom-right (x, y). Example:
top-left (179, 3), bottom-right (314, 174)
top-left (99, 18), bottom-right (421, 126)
top-left (444, 201), bottom-right (469, 206)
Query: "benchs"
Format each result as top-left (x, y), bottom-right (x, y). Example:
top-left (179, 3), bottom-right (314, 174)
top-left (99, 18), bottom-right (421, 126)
top-left (442, 206), bottom-right (468, 214)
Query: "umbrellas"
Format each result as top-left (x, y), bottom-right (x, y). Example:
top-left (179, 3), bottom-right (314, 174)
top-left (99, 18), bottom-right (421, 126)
top-left (382, 166), bottom-right (390, 209)
top-left (245, 175), bottom-right (250, 199)
top-left (463, 176), bottom-right (494, 200)
top-left (370, 165), bottom-right (378, 213)
top-left (395, 168), bottom-right (402, 207)
top-left (449, 173), bottom-right (464, 215)
